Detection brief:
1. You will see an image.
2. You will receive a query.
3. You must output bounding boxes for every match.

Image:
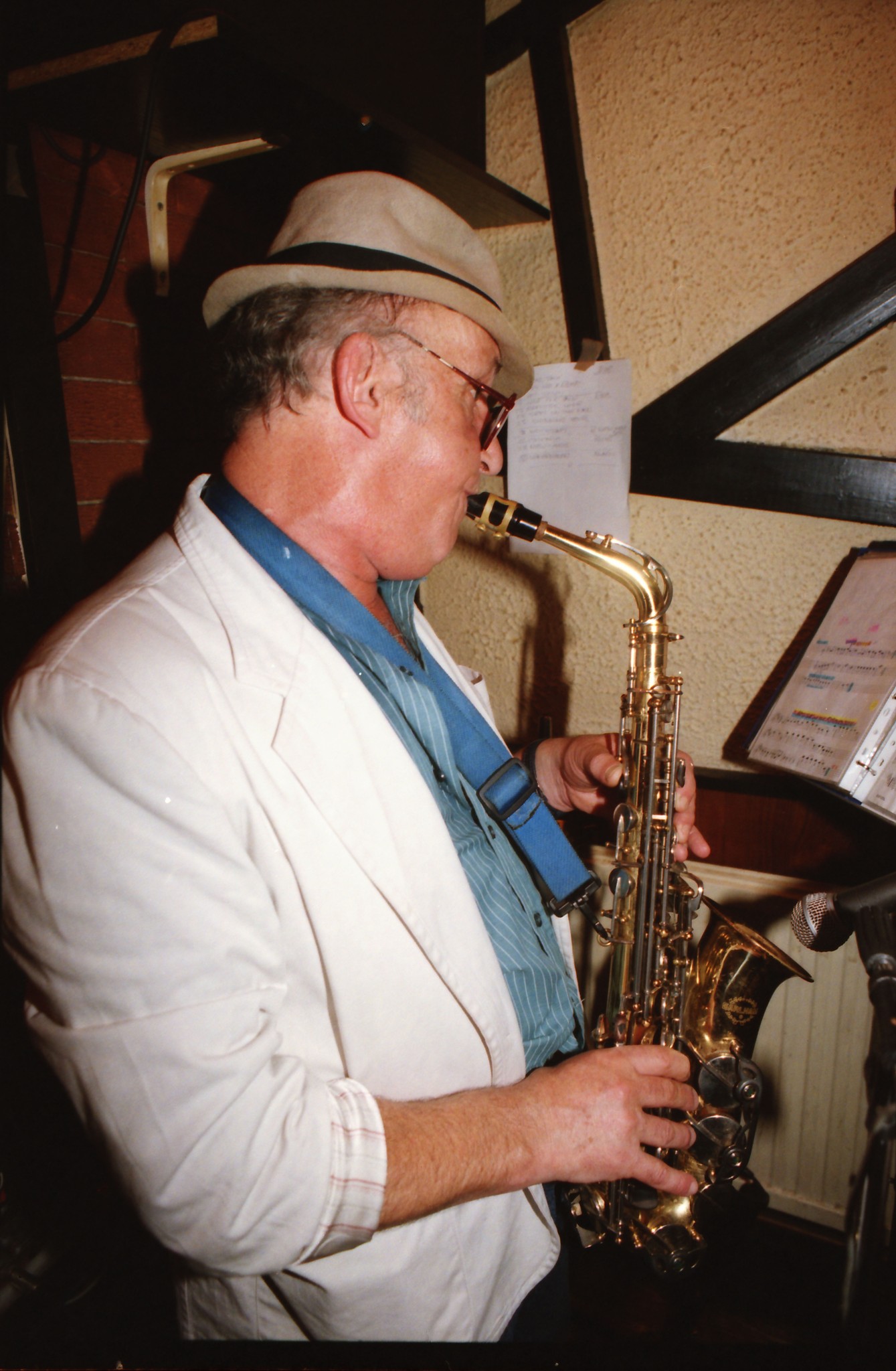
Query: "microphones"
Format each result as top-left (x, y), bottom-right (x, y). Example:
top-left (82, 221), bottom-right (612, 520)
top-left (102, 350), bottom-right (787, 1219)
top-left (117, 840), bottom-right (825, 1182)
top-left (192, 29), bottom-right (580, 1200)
top-left (790, 872), bottom-right (896, 953)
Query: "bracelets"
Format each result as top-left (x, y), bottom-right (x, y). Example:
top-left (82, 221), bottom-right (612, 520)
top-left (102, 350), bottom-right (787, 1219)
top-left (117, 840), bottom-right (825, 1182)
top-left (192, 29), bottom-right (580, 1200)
top-left (522, 715), bottom-right (578, 818)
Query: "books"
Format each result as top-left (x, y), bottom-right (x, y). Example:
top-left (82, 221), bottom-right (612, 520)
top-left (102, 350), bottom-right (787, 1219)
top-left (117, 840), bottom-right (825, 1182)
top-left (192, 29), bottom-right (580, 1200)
top-left (725, 547), bottom-right (896, 827)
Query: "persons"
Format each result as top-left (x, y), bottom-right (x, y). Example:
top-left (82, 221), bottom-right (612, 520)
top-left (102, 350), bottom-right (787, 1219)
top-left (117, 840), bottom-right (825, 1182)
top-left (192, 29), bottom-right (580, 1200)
top-left (0, 169), bottom-right (708, 1346)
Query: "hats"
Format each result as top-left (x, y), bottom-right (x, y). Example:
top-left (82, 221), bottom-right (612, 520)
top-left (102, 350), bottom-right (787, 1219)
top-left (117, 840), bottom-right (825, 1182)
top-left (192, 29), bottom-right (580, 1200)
top-left (203, 171), bottom-right (533, 408)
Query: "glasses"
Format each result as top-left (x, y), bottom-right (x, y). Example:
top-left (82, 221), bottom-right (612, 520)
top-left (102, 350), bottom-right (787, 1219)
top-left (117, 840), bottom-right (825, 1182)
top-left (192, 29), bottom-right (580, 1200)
top-left (375, 330), bottom-right (517, 450)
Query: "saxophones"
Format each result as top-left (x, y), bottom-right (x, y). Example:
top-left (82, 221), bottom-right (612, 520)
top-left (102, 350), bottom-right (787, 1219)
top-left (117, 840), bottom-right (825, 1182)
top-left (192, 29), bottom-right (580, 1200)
top-left (464, 485), bottom-right (816, 1276)
